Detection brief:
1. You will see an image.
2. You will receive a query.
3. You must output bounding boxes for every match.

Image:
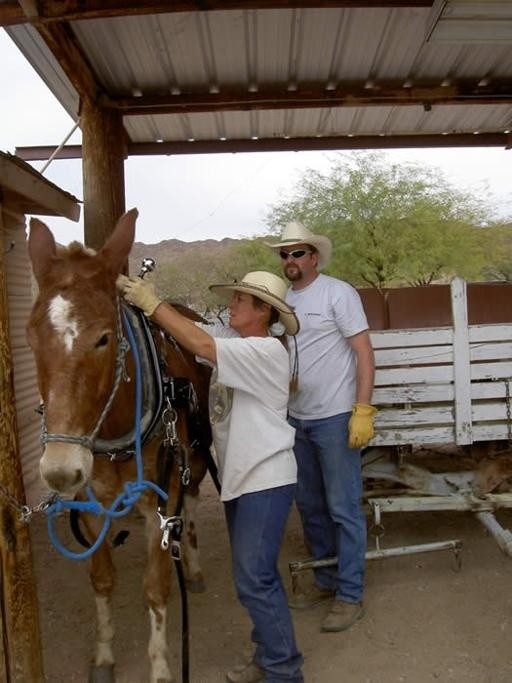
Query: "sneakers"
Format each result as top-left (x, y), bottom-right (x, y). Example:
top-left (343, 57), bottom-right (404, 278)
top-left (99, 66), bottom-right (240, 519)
top-left (288, 585), bottom-right (335, 609)
top-left (226, 658), bottom-right (264, 682)
top-left (322, 597), bottom-right (367, 632)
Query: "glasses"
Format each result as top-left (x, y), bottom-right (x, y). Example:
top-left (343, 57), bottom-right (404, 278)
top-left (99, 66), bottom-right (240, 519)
top-left (279, 248), bottom-right (312, 260)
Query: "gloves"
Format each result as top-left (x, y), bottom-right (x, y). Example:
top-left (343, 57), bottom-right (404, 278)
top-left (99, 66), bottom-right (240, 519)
top-left (346, 402), bottom-right (378, 448)
top-left (115, 272), bottom-right (162, 316)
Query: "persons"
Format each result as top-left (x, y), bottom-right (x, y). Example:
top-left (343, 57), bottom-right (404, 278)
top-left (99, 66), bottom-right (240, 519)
top-left (263, 218), bottom-right (379, 633)
top-left (122, 269), bottom-right (304, 681)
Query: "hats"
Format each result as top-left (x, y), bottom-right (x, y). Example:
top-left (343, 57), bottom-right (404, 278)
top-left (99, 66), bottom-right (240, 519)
top-left (262, 220), bottom-right (333, 272)
top-left (208, 271), bottom-right (302, 336)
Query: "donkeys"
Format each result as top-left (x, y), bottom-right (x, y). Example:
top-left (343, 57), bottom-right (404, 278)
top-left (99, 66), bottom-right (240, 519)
top-left (23, 207), bottom-right (213, 682)
